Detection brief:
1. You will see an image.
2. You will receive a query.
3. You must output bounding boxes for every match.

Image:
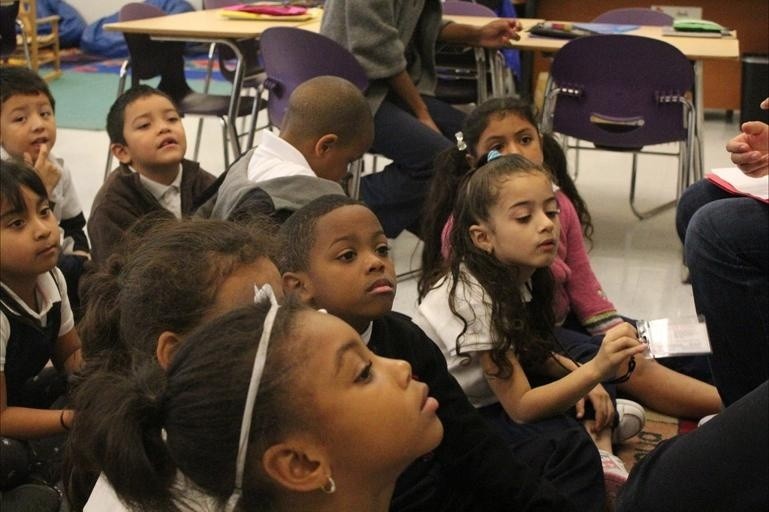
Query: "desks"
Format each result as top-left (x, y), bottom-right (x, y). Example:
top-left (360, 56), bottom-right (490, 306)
top-left (105, 0), bottom-right (740, 126)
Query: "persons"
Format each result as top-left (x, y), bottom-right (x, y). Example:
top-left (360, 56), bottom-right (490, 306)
top-left (76, 218), bottom-right (287, 512)
top-left (273, 193), bottom-right (477, 512)
top-left (0, 157), bottom-right (86, 512)
top-left (419, 95), bottom-right (725, 512)
top-left (673, 97), bottom-right (769, 408)
top-left (317, 0), bottom-right (524, 241)
top-left (190, 76), bottom-right (376, 233)
top-left (0, 64), bottom-right (92, 320)
top-left (59, 283), bottom-right (445, 512)
top-left (85, 85), bottom-right (219, 265)
top-left (410, 149), bottom-right (646, 510)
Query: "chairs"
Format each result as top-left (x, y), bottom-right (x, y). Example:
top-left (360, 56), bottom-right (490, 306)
top-left (194, 1), bottom-right (272, 160)
top-left (541, 33), bottom-right (705, 221)
top-left (435, 0), bottom-right (497, 108)
top-left (592, 8), bottom-right (673, 27)
top-left (256, 28), bottom-right (378, 201)
top-left (0, 0), bottom-right (20, 63)
top-left (100, 1), bottom-right (264, 182)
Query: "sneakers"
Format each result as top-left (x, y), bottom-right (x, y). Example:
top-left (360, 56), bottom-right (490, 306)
top-left (615, 399), bottom-right (646, 442)
top-left (600, 450), bottom-right (630, 487)
